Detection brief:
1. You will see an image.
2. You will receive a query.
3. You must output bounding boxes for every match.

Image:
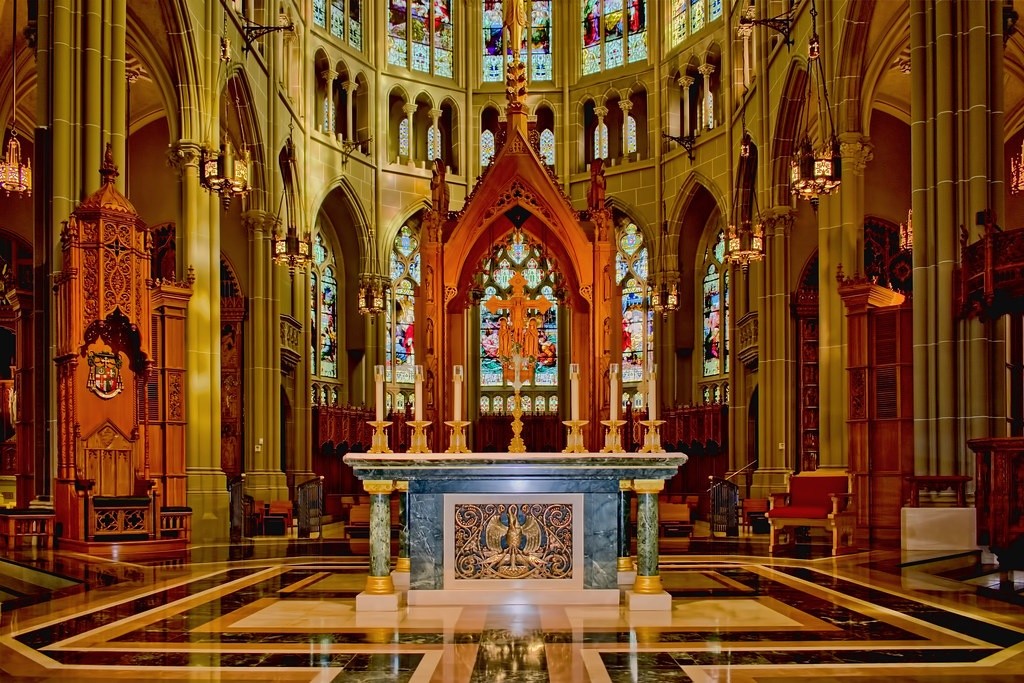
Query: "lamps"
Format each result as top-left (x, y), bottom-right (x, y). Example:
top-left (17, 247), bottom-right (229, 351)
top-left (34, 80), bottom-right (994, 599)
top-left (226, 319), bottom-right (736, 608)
top-left (899, 209), bottom-right (913, 256)
top-left (199, 0), bottom-right (394, 324)
top-left (647, 0), bottom-right (842, 321)
top-left (0, 0), bottom-right (32, 199)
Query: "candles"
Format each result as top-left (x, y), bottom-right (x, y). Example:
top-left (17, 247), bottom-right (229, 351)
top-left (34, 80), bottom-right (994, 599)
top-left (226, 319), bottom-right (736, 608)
top-left (373, 365), bottom-right (385, 422)
top-left (609, 364), bottom-right (618, 423)
top-left (570, 364), bottom-right (580, 421)
top-left (415, 364), bottom-right (423, 421)
top-left (648, 372), bottom-right (656, 421)
top-left (452, 364), bottom-right (463, 421)
top-left (514, 354), bottom-right (520, 382)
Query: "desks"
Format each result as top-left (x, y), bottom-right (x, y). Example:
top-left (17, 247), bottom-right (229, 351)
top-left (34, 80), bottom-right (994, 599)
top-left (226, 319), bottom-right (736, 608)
top-left (160, 506), bottom-right (193, 542)
top-left (342, 452), bottom-right (690, 612)
top-left (965, 437), bottom-right (1024, 592)
top-left (263, 515), bottom-right (283, 537)
top-left (903, 475), bottom-right (973, 508)
top-left (742, 499), bottom-right (770, 533)
top-left (0, 509), bottom-right (56, 550)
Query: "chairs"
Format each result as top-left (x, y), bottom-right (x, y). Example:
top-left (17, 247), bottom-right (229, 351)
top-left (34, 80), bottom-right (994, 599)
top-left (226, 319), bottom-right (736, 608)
top-left (768, 468), bottom-right (859, 556)
top-left (359, 496), bottom-right (370, 504)
top-left (74, 417), bottom-right (157, 543)
top-left (254, 501), bottom-right (293, 534)
top-left (657, 496), bottom-right (698, 509)
top-left (341, 497), bottom-right (354, 503)
